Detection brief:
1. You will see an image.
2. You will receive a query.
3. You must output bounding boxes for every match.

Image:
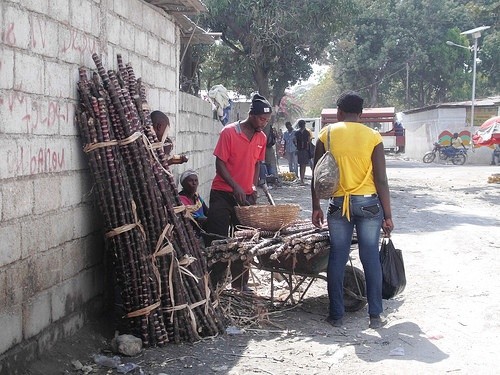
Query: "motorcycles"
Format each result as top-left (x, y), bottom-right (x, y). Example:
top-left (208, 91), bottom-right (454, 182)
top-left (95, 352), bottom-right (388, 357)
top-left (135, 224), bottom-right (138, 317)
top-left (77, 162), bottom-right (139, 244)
top-left (424, 139), bottom-right (470, 165)
top-left (446, 25), bottom-right (491, 163)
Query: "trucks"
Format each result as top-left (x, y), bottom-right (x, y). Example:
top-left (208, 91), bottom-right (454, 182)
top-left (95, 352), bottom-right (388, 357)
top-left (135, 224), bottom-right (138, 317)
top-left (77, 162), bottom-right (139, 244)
top-left (291, 117), bottom-right (397, 152)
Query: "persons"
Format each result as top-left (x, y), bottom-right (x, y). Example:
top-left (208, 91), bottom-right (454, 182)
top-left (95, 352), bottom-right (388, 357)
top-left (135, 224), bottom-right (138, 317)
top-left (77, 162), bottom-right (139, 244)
top-left (273, 127), bottom-right (283, 143)
top-left (149, 111), bottom-right (189, 166)
top-left (310, 90), bottom-right (394, 327)
top-left (205, 94), bottom-right (272, 291)
top-left (441, 133), bottom-right (466, 160)
top-left (282, 122), bottom-right (298, 178)
top-left (490, 144), bottom-right (500, 165)
top-left (293, 120), bottom-right (313, 185)
top-left (384, 121), bottom-right (405, 153)
top-left (178, 169), bottom-right (209, 243)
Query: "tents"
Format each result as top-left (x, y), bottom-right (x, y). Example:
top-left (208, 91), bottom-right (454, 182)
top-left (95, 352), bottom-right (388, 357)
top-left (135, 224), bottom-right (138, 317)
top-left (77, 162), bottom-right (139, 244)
top-left (472, 116), bottom-right (500, 148)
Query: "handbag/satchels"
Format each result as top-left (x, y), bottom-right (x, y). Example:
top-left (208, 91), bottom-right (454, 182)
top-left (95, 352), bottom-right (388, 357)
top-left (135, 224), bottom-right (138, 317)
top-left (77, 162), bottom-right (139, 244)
top-left (314, 126), bottom-right (337, 199)
top-left (380, 232), bottom-right (406, 300)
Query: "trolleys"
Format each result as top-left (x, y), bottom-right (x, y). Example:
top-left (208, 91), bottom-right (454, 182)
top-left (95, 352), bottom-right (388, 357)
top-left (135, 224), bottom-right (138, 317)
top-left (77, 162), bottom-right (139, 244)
top-left (210, 225), bottom-right (368, 313)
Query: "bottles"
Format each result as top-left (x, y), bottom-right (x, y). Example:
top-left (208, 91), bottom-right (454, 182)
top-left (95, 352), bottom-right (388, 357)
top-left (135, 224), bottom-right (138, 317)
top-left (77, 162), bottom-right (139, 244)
top-left (93, 355), bottom-right (121, 368)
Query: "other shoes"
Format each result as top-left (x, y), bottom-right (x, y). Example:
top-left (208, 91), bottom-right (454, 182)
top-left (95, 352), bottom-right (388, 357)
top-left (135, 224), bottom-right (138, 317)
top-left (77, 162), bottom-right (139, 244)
top-left (369, 316), bottom-right (388, 328)
top-left (326, 316), bottom-right (342, 327)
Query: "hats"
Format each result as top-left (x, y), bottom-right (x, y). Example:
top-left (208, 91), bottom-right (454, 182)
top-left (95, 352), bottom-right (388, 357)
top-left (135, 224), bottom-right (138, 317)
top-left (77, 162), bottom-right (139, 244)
top-left (285, 121), bottom-right (292, 126)
top-left (249, 93), bottom-right (273, 114)
top-left (336, 90), bottom-right (363, 112)
